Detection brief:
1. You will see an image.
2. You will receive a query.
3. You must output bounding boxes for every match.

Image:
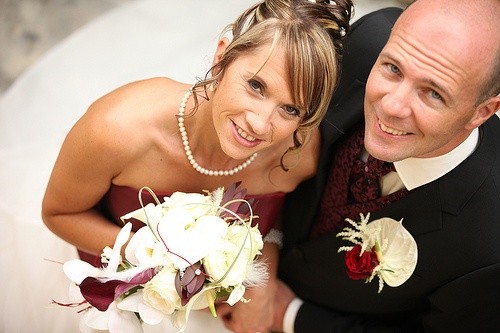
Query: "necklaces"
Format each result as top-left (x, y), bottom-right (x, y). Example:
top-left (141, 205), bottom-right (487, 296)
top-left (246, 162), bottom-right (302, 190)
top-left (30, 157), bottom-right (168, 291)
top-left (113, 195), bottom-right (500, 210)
top-left (179, 84), bottom-right (260, 178)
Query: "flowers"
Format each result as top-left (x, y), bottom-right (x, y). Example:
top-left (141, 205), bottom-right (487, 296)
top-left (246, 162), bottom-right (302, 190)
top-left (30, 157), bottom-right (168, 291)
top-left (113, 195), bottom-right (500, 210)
top-left (336, 212), bottom-right (419, 293)
top-left (51, 181), bottom-right (270, 333)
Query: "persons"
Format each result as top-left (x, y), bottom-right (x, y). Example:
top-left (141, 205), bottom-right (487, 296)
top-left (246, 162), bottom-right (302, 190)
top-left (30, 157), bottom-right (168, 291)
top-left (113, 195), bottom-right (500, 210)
top-left (213, 0), bottom-right (500, 333)
top-left (41, 0), bottom-right (356, 333)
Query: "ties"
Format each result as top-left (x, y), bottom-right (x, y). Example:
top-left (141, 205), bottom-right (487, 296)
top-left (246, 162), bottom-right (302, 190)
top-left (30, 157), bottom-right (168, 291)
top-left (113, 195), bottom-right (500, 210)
top-left (350, 154), bottom-right (396, 202)
